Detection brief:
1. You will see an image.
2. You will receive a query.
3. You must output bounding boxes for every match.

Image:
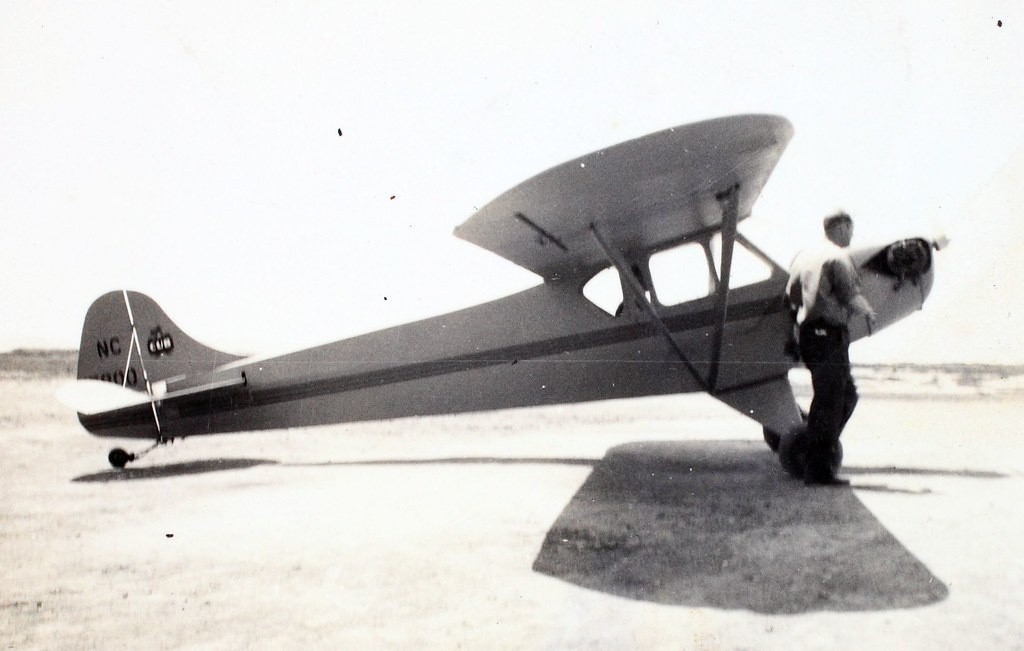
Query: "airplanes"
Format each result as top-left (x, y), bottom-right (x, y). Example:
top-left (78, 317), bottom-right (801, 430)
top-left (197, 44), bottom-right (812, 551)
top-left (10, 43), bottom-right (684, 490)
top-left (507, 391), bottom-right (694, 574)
top-left (75, 111), bottom-right (949, 482)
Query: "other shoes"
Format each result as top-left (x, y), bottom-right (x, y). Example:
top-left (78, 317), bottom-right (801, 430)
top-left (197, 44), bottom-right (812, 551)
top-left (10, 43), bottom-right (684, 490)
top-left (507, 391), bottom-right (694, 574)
top-left (805, 476), bottom-right (850, 486)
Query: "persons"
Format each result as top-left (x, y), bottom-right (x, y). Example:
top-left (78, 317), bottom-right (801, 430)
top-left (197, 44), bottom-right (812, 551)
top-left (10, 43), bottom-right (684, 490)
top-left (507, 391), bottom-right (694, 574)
top-left (783, 210), bottom-right (877, 488)
